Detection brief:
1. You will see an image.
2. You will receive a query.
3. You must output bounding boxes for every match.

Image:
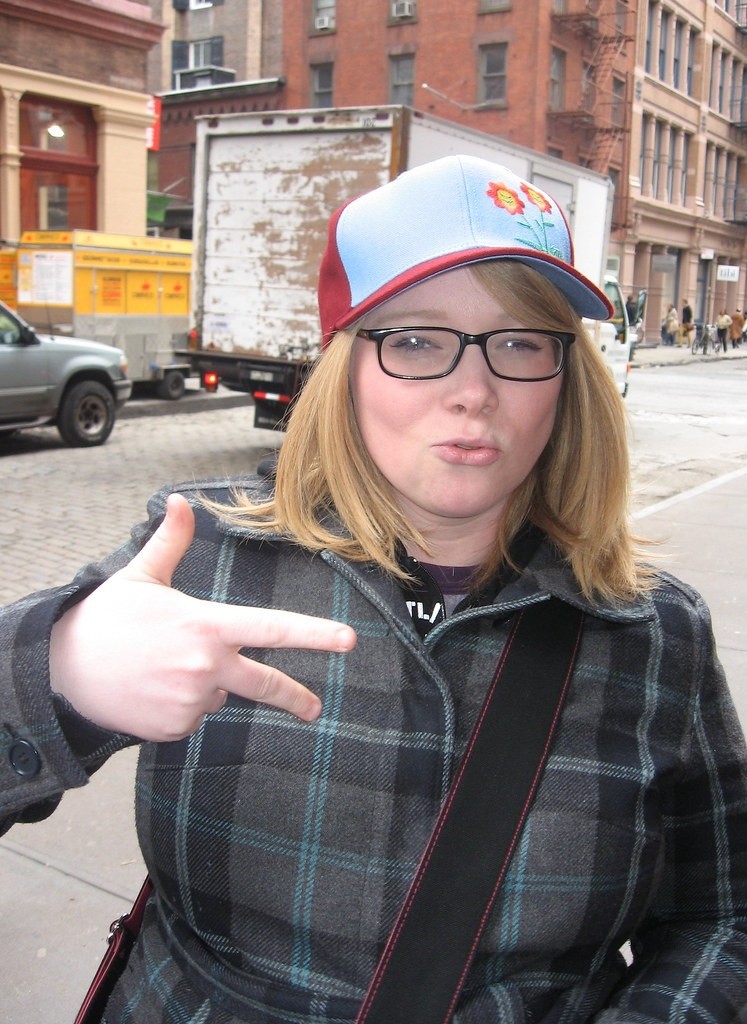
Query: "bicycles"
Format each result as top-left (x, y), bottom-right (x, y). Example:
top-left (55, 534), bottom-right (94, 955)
top-left (691, 322), bottom-right (716, 357)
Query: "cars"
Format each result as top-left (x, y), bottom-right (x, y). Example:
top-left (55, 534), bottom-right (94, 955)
top-left (0, 298), bottom-right (136, 448)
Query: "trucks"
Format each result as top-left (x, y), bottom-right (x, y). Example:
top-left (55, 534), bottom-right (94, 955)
top-left (1, 227), bottom-right (200, 400)
top-left (189, 104), bottom-right (648, 437)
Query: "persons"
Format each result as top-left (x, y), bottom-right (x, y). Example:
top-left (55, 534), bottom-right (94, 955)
top-left (664, 299), bottom-right (747, 352)
top-left (0, 152), bottom-right (747, 1024)
top-left (626, 296), bottom-right (643, 337)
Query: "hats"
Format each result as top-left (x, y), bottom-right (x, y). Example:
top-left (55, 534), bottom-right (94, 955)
top-left (316, 154), bottom-right (617, 348)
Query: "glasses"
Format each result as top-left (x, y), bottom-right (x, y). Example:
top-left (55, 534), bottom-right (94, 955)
top-left (357, 326), bottom-right (575, 382)
